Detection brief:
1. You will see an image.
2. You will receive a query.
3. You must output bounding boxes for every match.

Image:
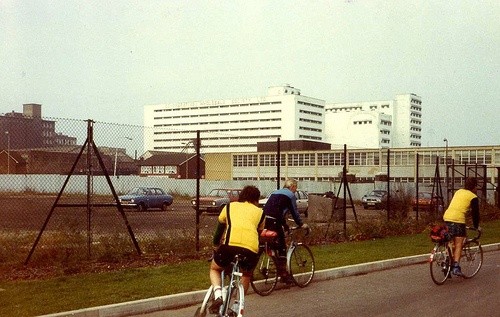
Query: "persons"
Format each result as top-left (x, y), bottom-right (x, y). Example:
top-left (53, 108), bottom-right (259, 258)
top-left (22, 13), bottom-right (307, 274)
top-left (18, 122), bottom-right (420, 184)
top-left (208, 185), bottom-right (266, 317)
top-left (263, 177), bottom-right (310, 283)
top-left (443, 177), bottom-right (482, 280)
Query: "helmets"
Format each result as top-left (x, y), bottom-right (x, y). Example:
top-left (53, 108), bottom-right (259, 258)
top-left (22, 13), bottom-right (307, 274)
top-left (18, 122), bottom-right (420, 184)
top-left (430, 225), bottom-right (450, 243)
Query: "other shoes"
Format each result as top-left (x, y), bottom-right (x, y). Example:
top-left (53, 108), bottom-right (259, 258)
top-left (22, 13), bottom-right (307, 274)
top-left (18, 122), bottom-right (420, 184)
top-left (280, 273), bottom-right (295, 283)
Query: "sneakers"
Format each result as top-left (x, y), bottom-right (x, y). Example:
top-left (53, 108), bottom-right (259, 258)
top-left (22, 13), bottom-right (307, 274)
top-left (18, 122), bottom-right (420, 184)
top-left (208, 296), bottom-right (223, 313)
top-left (443, 266), bottom-right (452, 279)
top-left (452, 266), bottom-right (465, 276)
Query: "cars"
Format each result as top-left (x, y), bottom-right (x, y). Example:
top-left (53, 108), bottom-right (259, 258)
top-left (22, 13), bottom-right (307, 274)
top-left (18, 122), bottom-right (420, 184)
top-left (362, 190), bottom-right (390, 209)
top-left (259, 190), bottom-right (309, 216)
top-left (411, 192), bottom-right (442, 211)
top-left (117, 187), bottom-right (173, 212)
top-left (192, 189), bottom-right (243, 215)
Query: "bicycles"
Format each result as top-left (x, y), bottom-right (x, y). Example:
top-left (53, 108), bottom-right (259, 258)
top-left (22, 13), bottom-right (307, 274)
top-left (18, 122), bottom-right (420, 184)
top-left (250, 225), bottom-right (315, 296)
top-left (200, 254), bottom-right (244, 317)
top-left (429, 226), bottom-right (483, 285)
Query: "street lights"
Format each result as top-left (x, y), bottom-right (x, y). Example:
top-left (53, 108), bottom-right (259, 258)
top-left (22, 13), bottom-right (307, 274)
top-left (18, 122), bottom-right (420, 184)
top-left (182, 142), bottom-right (192, 179)
top-left (5, 130), bottom-right (11, 176)
top-left (444, 138), bottom-right (448, 169)
top-left (114, 135), bottom-right (133, 176)
